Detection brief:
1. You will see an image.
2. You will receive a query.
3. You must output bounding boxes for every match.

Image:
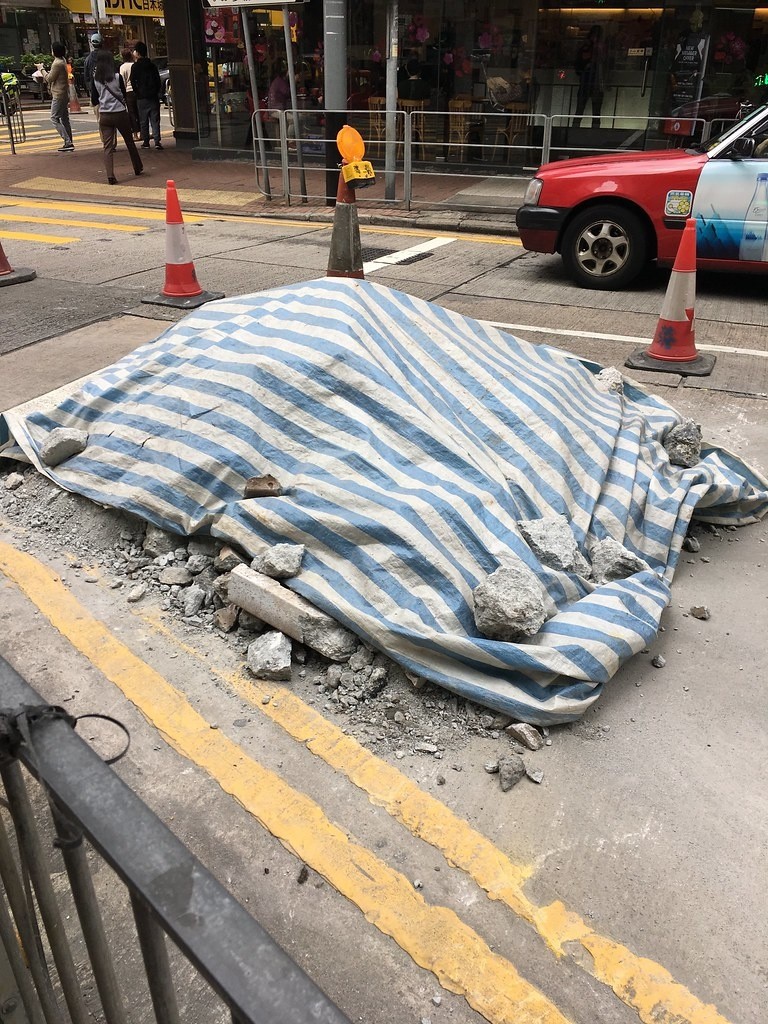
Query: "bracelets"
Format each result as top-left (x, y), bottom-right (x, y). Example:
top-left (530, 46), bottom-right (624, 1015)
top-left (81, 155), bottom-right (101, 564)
top-left (41, 68), bottom-right (45, 73)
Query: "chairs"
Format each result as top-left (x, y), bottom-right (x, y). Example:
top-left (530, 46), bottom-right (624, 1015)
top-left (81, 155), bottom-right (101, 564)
top-left (246, 88), bottom-right (283, 151)
top-left (318, 92), bottom-right (362, 127)
top-left (366, 96), bottom-right (537, 166)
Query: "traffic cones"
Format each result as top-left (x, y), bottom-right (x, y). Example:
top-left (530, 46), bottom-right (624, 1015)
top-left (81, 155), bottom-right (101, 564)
top-left (325, 159), bottom-right (366, 281)
top-left (141, 180), bottom-right (225, 311)
top-left (0, 238), bottom-right (38, 289)
top-left (624, 218), bottom-right (718, 378)
top-left (67, 79), bottom-right (89, 115)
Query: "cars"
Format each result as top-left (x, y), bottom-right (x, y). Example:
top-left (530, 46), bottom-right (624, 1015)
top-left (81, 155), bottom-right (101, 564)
top-left (515, 95), bottom-right (768, 289)
top-left (148, 55), bottom-right (175, 105)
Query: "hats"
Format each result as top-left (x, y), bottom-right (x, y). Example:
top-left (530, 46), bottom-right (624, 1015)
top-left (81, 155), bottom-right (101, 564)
top-left (91, 33), bottom-right (104, 44)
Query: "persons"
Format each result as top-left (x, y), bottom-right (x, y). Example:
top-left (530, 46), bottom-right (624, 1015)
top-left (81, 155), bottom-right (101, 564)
top-left (266, 64), bottom-right (301, 151)
top-left (571, 24), bottom-right (615, 128)
top-left (292, 41), bottom-right (309, 72)
top-left (34, 43), bottom-right (75, 152)
top-left (84, 33), bottom-right (162, 184)
top-left (68, 56), bottom-right (83, 98)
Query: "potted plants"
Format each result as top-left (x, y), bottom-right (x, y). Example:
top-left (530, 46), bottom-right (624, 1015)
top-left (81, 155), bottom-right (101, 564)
top-left (73, 54), bottom-right (125, 92)
top-left (0, 55), bottom-right (17, 73)
top-left (20, 53), bottom-right (55, 94)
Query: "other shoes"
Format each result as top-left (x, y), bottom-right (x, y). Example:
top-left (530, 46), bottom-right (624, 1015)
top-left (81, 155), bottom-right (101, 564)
top-left (108, 176), bottom-right (118, 184)
top-left (288, 143), bottom-right (297, 152)
top-left (135, 167), bottom-right (143, 175)
top-left (141, 142), bottom-right (150, 148)
top-left (156, 143), bottom-right (164, 150)
top-left (58, 144), bottom-right (75, 152)
top-left (113, 145), bottom-right (116, 152)
